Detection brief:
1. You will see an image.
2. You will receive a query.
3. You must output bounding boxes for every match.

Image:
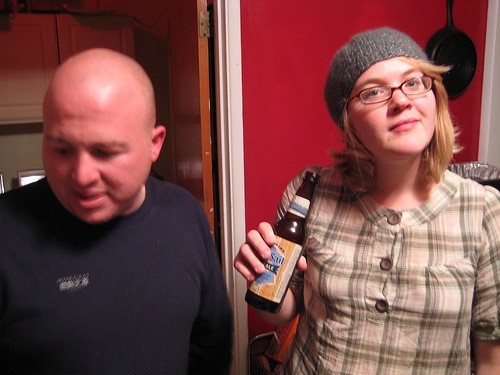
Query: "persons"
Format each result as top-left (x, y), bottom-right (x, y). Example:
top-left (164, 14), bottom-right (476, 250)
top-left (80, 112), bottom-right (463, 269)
top-left (0, 49), bottom-right (233, 375)
top-left (231, 27), bottom-right (500, 375)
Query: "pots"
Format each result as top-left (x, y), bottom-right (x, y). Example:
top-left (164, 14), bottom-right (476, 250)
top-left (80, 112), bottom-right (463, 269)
top-left (422, 0), bottom-right (478, 100)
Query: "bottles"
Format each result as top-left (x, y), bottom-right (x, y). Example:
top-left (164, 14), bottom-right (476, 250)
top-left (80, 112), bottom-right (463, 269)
top-left (245, 170), bottom-right (322, 313)
